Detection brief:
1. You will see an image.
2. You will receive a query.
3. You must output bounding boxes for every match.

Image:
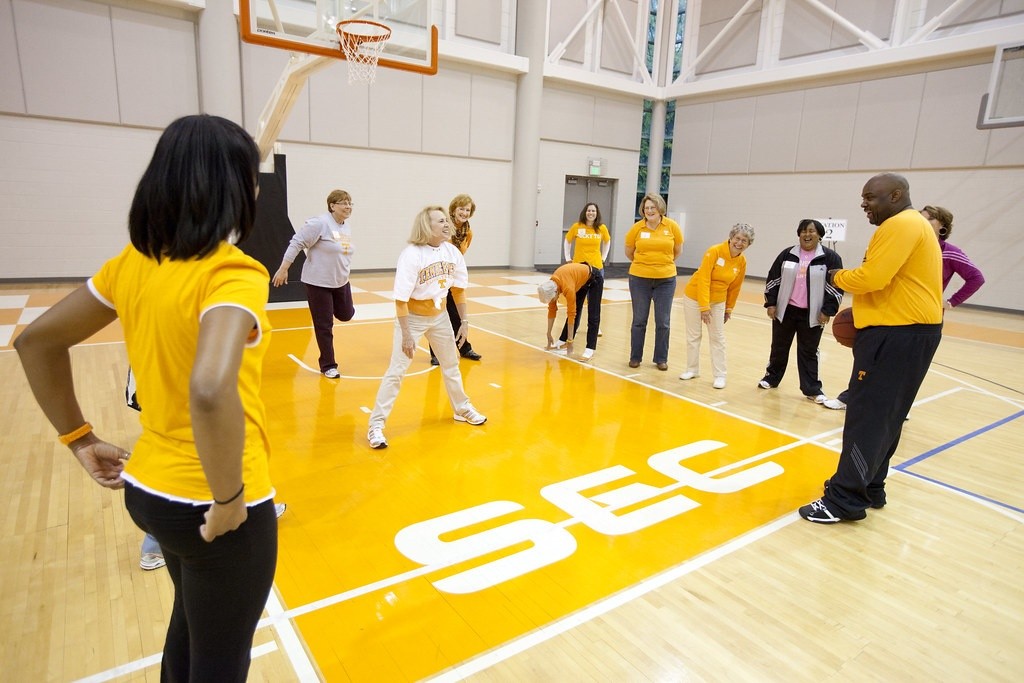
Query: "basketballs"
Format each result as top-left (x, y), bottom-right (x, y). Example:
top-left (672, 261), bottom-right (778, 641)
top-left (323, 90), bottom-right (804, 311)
top-left (832, 307), bottom-right (858, 349)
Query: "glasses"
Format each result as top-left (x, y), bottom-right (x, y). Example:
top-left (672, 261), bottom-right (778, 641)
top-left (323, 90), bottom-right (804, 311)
top-left (333, 201), bottom-right (355, 207)
top-left (732, 236), bottom-right (750, 244)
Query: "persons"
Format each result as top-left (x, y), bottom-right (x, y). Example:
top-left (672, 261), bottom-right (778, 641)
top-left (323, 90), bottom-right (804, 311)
top-left (798, 172), bottom-right (942, 524)
top-left (680, 222), bottom-right (756, 388)
top-left (272, 190), bottom-right (356, 378)
top-left (823, 202), bottom-right (986, 422)
top-left (537, 263), bottom-right (604, 361)
top-left (545, 203), bottom-right (612, 350)
top-left (625, 192), bottom-right (684, 372)
top-left (14, 112), bottom-right (278, 683)
top-left (428, 194), bottom-right (483, 364)
top-left (366, 206), bottom-right (488, 450)
top-left (758, 220), bottom-right (843, 403)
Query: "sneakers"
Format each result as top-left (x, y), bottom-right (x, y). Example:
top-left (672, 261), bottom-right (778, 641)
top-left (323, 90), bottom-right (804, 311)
top-left (679, 370), bottom-right (700, 380)
top-left (806, 394), bottom-right (828, 404)
top-left (431, 357), bottom-right (439, 365)
top-left (274, 501), bottom-right (287, 519)
top-left (823, 479), bottom-right (887, 509)
top-left (367, 426), bottom-right (389, 449)
top-left (453, 403), bottom-right (487, 425)
top-left (798, 498), bottom-right (867, 524)
top-left (140, 552), bottom-right (168, 570)
top-left (823, 398), bottom-right (847, 410)
top-left (460, 349), bottom-right (482, 361)
top-left (543, 339), bottom-right (567, 350)
top-left (579, 347), bottom-right (594, 362)
top-left (757, 380), bottom-right (773, 389)
top-left (712, 376), bottom-right (727, 389)
top-left (322, 368), bottom-right (340, 378)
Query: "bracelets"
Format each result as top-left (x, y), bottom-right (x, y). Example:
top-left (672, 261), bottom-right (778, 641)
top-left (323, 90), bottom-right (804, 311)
top-left (214, 483), bottom-right (245, 504)
top-left (460, 319), bottom-right (469, 322)
top-left (566, 339), bottom-right (574, 343)
top-left (59, 423), bottom-right (93, 446)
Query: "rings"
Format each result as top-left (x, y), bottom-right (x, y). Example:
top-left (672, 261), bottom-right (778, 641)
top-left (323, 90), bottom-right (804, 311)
top-left (817, 319), bottom-right (819, 320)
top-left (125, 453), bottom-right (130, 459)
top-left (825, 321), bottom-right (828, 324)
top-left (822, 321), bottom-right (825, 324)
top-left (110, 479), bottom-right (114, 481)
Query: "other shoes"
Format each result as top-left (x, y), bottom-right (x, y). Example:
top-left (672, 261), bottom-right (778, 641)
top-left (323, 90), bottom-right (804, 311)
top-left (597, 328), bottom-right (603, 337)
top-left (656, 362), bottom-right (668, 371)
top-left (629, 360), bottom-right (640, 368)
